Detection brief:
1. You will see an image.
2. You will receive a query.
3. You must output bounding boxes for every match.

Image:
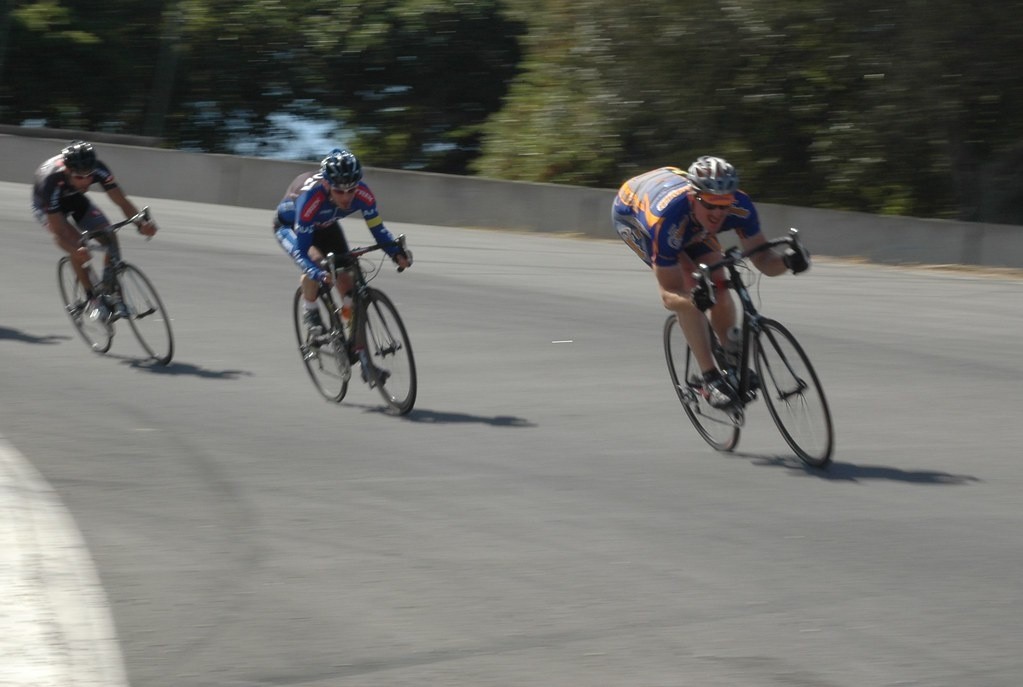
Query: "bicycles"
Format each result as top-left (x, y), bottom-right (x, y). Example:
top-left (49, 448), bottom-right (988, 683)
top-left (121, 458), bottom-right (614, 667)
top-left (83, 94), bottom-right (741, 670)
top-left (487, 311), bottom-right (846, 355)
top-left (55, 205), bottom-right (174, 364)
top-left (292, 233), bottom-right (419, 416)
top-left (661, 227), bottom-right (835, 469)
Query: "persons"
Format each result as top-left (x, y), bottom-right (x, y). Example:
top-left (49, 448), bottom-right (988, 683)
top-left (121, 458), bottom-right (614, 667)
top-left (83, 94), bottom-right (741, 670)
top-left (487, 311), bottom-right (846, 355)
top-left (272, 151), bottom-right (413, 382)
top-left (612, 155), bottom-right (810, 406)
top-left (31, 141), bottom-right (158, 319)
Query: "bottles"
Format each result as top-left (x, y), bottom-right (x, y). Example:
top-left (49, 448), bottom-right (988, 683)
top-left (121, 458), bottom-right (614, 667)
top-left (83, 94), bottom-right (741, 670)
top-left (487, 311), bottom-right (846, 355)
top-left (341, 288), bottom-right (353, 321)
top-left (331, 283), bottom-right (347, 323)
top-left (724, 327), bottom-right (741, 366)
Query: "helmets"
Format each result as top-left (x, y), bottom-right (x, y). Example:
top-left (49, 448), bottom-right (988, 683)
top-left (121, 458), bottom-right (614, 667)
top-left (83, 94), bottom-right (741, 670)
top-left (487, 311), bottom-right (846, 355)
top-left (687, 156), bottom-right (738, 197)
top-left (62, 140), bottom-right (96, 172)
top-left (321, 149), bottom-right (363, 190)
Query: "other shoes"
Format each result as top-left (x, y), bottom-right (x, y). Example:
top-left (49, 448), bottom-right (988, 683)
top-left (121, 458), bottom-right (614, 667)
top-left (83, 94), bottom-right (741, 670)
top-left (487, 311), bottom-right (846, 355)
top-left (702, 380), bottom-right (738, 410)
top-left (301, 306), bottom-right (325, 335)
top-left (360, 365), bottom-right (390, 380)
top-left (729, 365), bottom-right (760, 389)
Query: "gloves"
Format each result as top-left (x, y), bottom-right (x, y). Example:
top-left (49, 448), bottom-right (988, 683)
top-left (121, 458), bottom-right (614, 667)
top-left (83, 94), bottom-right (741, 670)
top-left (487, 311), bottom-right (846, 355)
top-left (690, 286), bottom-right (715, 312)
top-left (783, 249), bottom-right (810, 272)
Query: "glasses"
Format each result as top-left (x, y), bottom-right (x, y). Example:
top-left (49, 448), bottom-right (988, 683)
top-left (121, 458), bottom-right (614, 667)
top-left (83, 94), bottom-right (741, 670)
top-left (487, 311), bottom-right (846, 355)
top-left (695, 193), bottom-right (732, 210)
top-left (329, 186), bottom-right (357, 195)
top-left (71, 169), bottom-right (97, 180)
